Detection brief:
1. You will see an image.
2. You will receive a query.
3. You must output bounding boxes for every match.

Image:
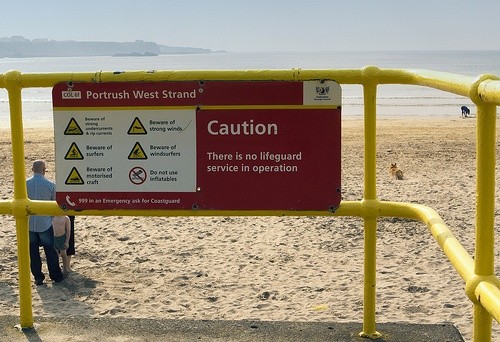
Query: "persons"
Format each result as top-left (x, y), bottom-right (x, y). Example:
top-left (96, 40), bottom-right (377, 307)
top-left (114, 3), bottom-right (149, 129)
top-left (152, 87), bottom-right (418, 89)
top-left (67, 216), bottom-right (75, 272)
top-left (26, 160), bottom-right (69, 286)
top-left (52, 215), bottom-right (70, 274)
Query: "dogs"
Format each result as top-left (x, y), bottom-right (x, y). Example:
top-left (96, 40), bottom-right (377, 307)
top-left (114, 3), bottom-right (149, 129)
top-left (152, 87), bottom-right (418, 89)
top-left (388, 162), bottom-right (403, 180)
top-left (461, 106), bottom-right (470, 117)
